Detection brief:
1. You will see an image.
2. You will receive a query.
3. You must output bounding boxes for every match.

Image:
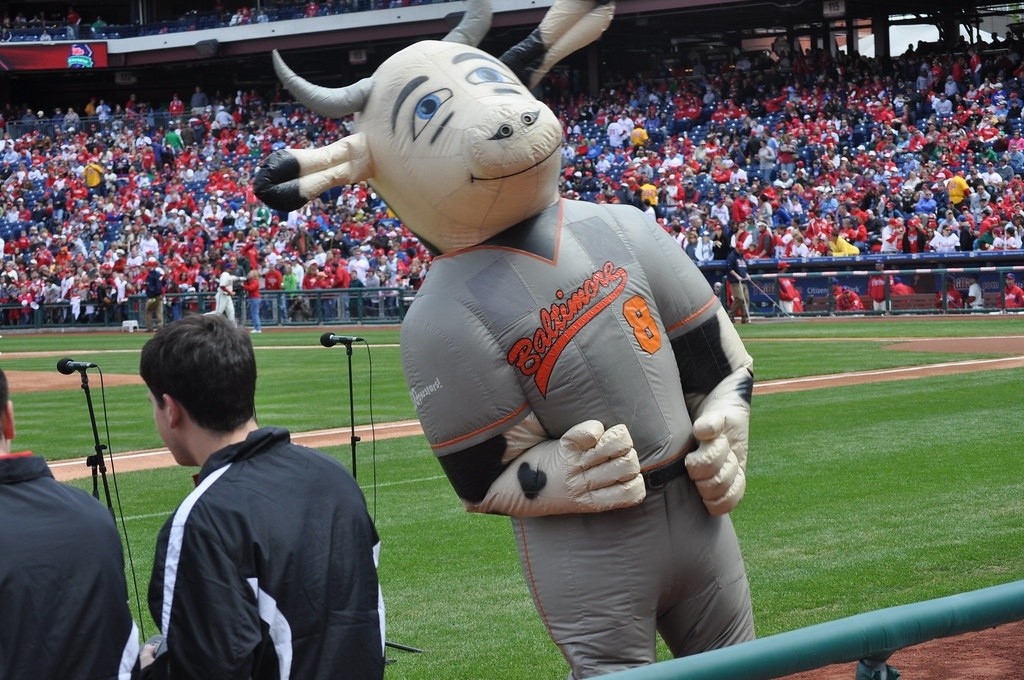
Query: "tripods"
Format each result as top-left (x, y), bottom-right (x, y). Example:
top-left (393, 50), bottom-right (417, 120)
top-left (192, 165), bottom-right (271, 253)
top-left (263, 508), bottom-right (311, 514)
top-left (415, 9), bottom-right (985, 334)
top-left (333, 348), bottom-right (421, 662)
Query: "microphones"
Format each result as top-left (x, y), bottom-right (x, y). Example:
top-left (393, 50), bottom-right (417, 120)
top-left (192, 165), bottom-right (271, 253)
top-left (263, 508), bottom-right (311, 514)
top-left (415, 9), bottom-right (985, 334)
top-left (320, 332), bottom-right (364, 347)
top-left (56, 358), bottom-right (96, 375)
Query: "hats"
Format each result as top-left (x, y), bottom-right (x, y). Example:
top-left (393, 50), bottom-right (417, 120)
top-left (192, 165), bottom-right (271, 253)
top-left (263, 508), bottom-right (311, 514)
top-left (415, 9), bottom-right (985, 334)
top-left (0, 55), bottom-right (1024, 304)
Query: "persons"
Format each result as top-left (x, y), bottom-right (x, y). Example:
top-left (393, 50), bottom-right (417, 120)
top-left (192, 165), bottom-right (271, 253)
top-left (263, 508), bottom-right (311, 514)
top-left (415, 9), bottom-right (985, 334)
top-left (123, 311), bottom-right (387, 680)
top-left (1, 0), bottom-right (1024, 324)
top-left (0, 364), bottom-right (144, 680)
